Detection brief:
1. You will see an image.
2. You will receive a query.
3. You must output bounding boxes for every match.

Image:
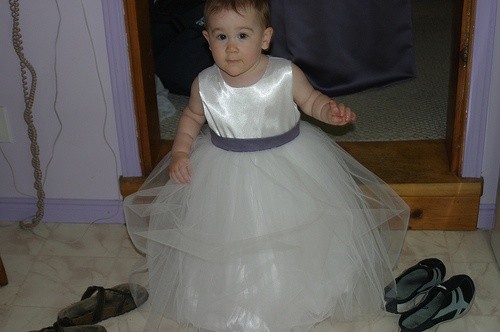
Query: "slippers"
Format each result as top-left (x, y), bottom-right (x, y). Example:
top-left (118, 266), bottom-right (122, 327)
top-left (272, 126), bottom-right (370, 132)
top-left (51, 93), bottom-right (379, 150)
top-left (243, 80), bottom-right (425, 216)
top-left (57, 283), bottom-right (150, 326)
top-left (398, 274), bottom-right (476, 332)
top-left (380, 258), bottom-right (446, 314)
top-left (28, 317), bottom-right (108, 332)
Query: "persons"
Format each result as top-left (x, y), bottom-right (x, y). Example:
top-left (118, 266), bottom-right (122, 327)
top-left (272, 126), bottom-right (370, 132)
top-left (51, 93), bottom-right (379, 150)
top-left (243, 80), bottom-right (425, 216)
top-left (122, 1), bottom-right (411, 332)
